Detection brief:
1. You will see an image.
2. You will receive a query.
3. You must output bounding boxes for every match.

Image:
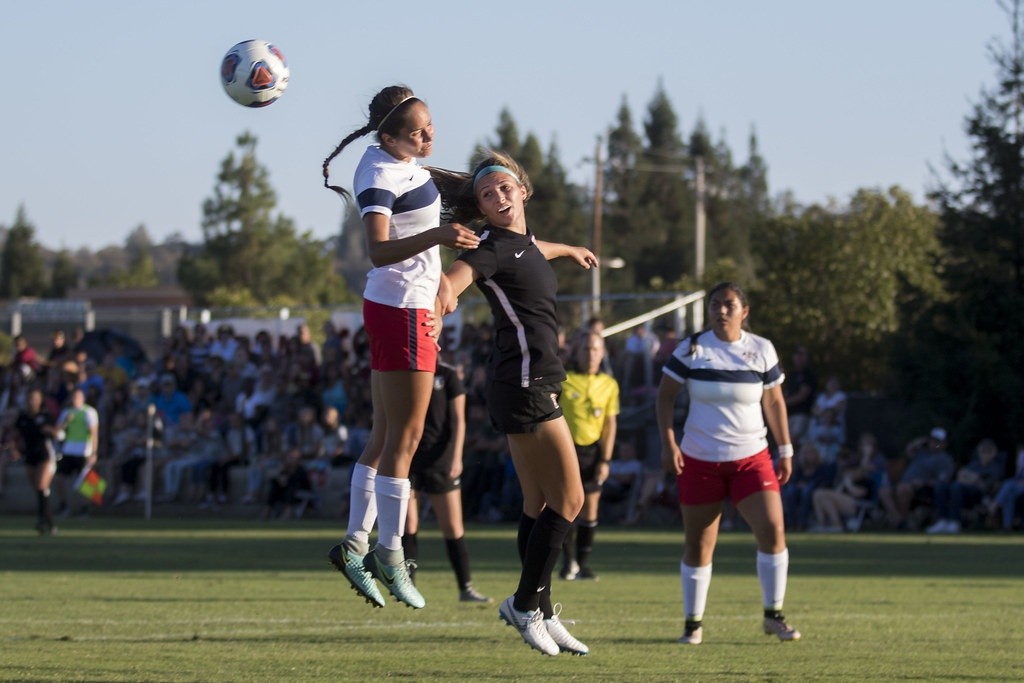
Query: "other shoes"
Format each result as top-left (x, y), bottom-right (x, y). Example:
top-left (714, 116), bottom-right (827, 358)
top-left (560, 560), bottom-right (579, 580)
top-left (806, 525), bottom-right (823, 532)
top-left (823, 525), bottom-right (843, 533)
top-left (925, 519), bottom-right (946, 534)
top-left (36, 520), bottom-right (52, 534)
top-left (460, 587), bottom-right (492, 602)
top-left (680, 626), bottom-right (702, 645)
top-left (763, 617), bottom-right (800, 641)
top-left (946, 520), bottom-right (961, 533)
top-left (579, 569), bottom-right (599, 581)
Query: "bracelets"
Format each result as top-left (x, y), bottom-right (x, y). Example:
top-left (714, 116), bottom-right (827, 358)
top-left (778, 444), bottom-right (794, 458)
top-left (599, 457), bottom-right (612, 466)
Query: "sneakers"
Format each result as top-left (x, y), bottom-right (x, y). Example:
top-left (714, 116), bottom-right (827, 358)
top-left (499, 595), bottom-right (560, 656)
top-left (362, 549), bottom-right (426, 609)
top-left (544, 603), bottom-right (589, 655)
top-left (328, 543), bottom-right (384, 609)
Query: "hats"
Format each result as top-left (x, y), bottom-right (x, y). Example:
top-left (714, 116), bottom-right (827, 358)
top-left (930, 427), bottom-right (946, 440)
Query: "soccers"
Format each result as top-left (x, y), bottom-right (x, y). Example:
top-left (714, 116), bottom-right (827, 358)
top-left (220, 37), bottom-right (290, 108)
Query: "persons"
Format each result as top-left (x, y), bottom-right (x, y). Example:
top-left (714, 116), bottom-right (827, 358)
top-left (656, 283), bottom-right (804, 646)
top-left (318, 82), bottom-right (481, 614)
top-left (403, 334), bottom-right (493, 604)
top-left (1, 310), bottom-right (1017, 534)
top-left (556, 329), bottom-right (622, 582)
top-left (422, 147), bottom-right (602, 659)
